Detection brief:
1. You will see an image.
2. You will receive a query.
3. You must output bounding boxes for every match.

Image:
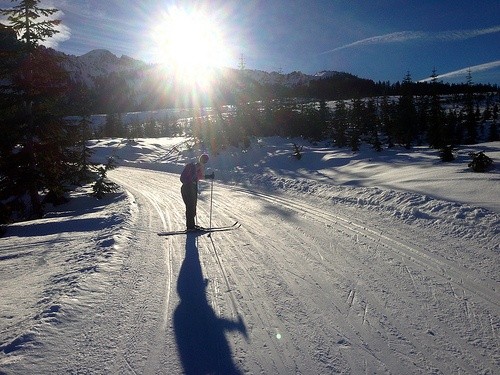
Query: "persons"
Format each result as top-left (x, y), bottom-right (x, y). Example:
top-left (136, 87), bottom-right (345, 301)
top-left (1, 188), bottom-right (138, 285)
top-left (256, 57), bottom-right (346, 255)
top-left (180, 155), bottom-right (209, 230)
top-left (174, 232), bottom-right (249, 375)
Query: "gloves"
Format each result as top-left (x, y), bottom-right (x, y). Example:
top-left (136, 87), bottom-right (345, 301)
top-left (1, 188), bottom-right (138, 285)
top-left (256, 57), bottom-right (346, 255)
top-left (205, 174), bottom-right (215, 179)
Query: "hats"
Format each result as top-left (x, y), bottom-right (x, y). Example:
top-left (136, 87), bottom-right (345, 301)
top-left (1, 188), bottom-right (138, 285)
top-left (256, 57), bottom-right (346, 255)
top-left (202, 153), bottom-right (210, 161)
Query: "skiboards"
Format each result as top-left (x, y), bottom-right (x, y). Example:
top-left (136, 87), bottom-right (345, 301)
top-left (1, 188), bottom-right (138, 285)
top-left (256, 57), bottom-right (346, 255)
top-left (158, 221), bottom-right (241, 235)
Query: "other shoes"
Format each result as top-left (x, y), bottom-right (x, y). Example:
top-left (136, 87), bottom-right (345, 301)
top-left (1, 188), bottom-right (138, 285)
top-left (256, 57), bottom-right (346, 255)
top-left (187, 224), bottom-right (200, 233)
